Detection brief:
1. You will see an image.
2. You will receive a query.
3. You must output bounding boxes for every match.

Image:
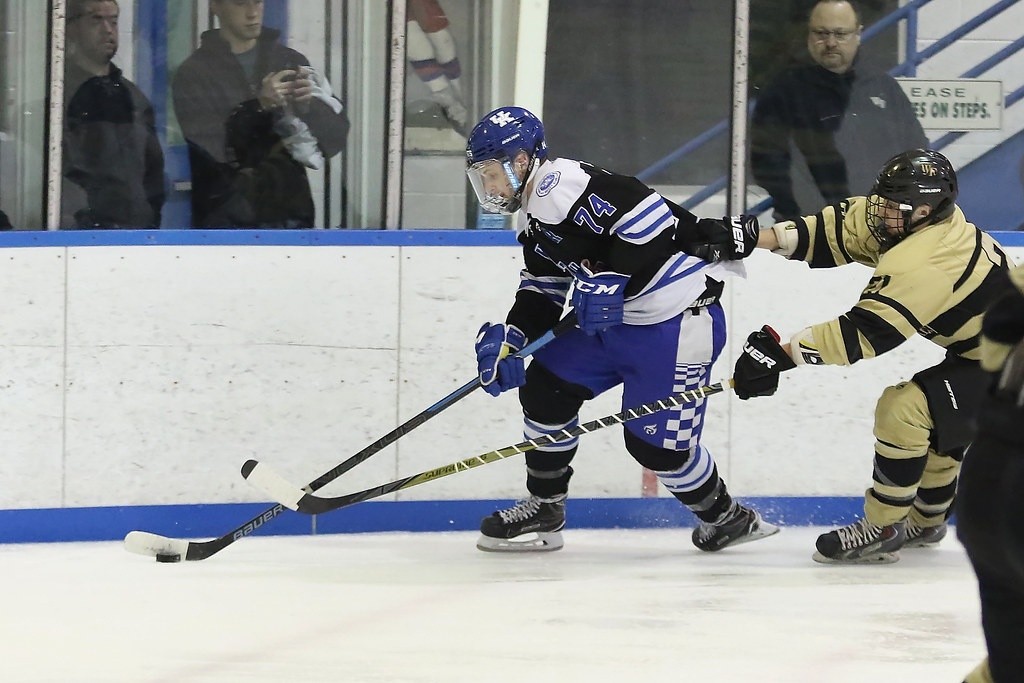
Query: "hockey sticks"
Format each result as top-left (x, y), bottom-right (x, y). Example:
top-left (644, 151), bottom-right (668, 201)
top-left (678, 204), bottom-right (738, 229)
top-left (121, 310), bottom-right (580, 562)
top-left (238, 377), bottom-right (738, 517)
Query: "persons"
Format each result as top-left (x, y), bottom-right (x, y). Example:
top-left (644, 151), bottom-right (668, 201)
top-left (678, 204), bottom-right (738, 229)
top-left (63, 0), bottom-right (168, 230)
top-left (464, 106), bottom-right (780, 551)
top-left (954, 265), bottom-right (1024, 682)
top-left (751, 0), bottom-right (929, 223)
top-left (693, 150), bottom-right (1024, 562)
top-left (173, 0), bottom-right (351, 230)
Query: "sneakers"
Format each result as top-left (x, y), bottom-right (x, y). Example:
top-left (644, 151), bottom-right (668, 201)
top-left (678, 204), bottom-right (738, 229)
top-left (902, 518), bottom-right (949, 548)
top-left (476, 492), bottom-right (566, 552)
top-left (812, 517), bottom-right (907, 564)
top-left (692, 499), bottom-right (780, 552)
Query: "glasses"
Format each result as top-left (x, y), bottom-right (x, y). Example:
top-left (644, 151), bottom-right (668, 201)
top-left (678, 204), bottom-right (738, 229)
top-left (809, 28), bottom-right (857, 44)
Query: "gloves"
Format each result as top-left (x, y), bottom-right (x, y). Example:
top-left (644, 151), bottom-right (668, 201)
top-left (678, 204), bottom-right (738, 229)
top-left (687, 214), bottom-right (760, 262)
top-left (733, 324), bottom-right (797, 400)
top-left (475, 322), bottom-right (526, 397)
top-left (572, 259), bottom-right (631, 336)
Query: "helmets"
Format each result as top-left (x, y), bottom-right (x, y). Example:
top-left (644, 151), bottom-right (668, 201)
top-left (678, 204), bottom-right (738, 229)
top-left (465, 107), bottom-right (547, 215)
top-left (865, 149), bottom-right (958, 252)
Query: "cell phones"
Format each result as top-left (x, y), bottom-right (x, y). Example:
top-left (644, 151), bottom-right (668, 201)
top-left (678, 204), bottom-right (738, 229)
top-left (278, 61), bottom-right (300, 83)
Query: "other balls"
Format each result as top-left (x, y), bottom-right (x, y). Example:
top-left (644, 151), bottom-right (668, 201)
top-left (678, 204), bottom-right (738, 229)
top-left (156, 552), bottom-right (182, 563)
